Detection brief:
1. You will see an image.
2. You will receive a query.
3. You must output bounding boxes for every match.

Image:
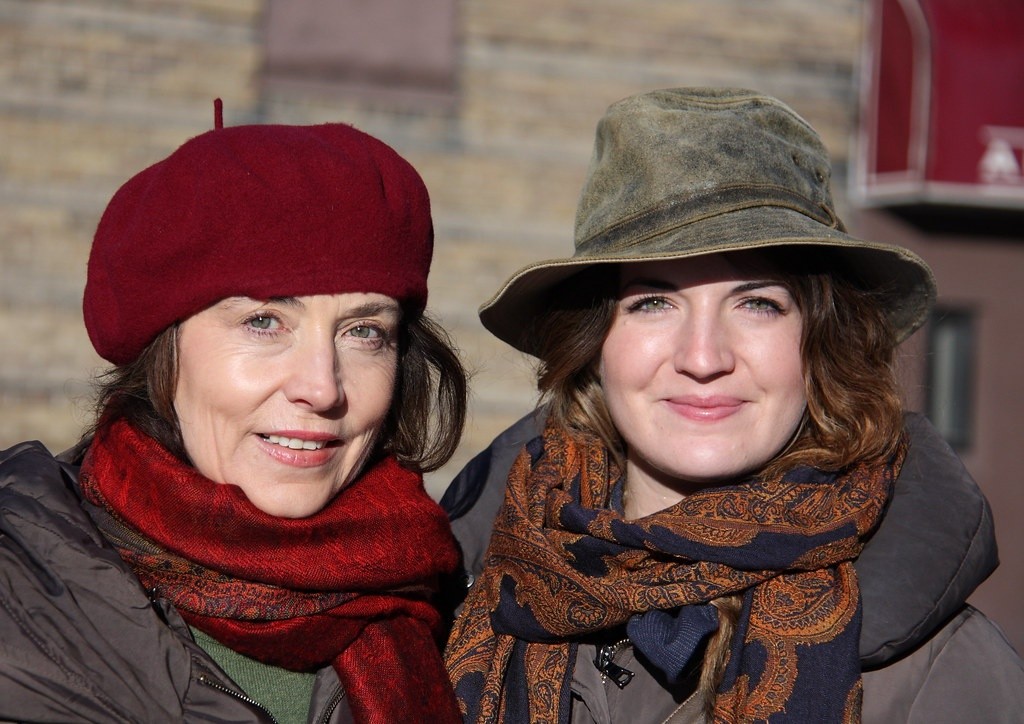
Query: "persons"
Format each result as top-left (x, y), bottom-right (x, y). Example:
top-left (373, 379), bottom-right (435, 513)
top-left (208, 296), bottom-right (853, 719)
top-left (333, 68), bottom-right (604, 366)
top-left (0, 93), bottom-right (471, 724)
top-left (447, 85), bottom-right (1024, 724)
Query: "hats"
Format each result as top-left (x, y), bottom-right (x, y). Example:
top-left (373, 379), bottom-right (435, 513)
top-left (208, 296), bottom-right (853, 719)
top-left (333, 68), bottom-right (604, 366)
top-left (82, 98), bottom-right (434, 367)
top-left (475, 86), bottom-right (938, 361)
